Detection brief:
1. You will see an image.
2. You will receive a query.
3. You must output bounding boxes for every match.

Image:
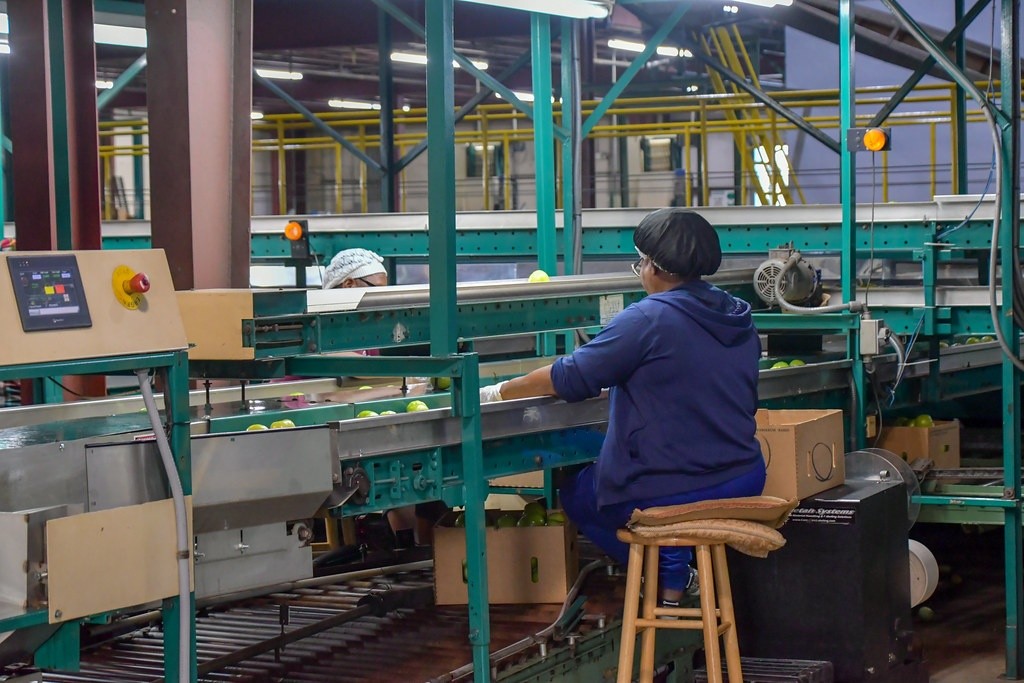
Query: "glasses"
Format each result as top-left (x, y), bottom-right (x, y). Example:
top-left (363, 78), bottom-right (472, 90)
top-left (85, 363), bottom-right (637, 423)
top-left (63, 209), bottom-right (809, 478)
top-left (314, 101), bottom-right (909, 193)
top-left (631, 258), bottom-right (643, 276)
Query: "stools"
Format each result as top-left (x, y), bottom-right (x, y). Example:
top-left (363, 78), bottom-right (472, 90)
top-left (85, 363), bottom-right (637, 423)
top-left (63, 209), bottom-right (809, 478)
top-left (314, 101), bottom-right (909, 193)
top-left (616, 529), bottom-right (743, 683)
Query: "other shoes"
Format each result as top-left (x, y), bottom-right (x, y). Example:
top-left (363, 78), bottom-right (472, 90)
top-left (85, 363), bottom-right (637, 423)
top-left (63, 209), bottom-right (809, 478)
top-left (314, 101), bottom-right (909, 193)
top-left (685, 565), bottom-right (701, 595)
top-left (640, 577), bottom-right (679, 620)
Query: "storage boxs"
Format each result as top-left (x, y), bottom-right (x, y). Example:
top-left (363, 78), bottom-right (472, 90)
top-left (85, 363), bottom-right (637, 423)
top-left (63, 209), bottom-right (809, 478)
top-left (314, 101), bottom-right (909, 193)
top-left (756, 408), bottom-right (846, 500)
top-left (871, 421), bottom-right (960, 484)
top-left (434, 516), bottom-right (577, 605)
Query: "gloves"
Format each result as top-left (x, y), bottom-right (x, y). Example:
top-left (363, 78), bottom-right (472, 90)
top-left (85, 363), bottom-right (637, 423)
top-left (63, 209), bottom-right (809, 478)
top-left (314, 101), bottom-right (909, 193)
top-left (479, 380), bottom-right (509, 403)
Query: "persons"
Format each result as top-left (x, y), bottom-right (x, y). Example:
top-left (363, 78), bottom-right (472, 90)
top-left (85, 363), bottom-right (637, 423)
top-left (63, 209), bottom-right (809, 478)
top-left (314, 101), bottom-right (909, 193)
top-left (478, 209), bottom-right (768, 617)
top-left (268, 245), bottom-right (449, 551)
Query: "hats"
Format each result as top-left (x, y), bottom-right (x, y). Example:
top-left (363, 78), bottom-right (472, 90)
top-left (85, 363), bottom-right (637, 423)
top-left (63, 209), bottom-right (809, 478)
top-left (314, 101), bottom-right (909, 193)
top-left (322, 248), bottom-right (387, 290)
top-left (633, 207), bottom-right (722, 276)
